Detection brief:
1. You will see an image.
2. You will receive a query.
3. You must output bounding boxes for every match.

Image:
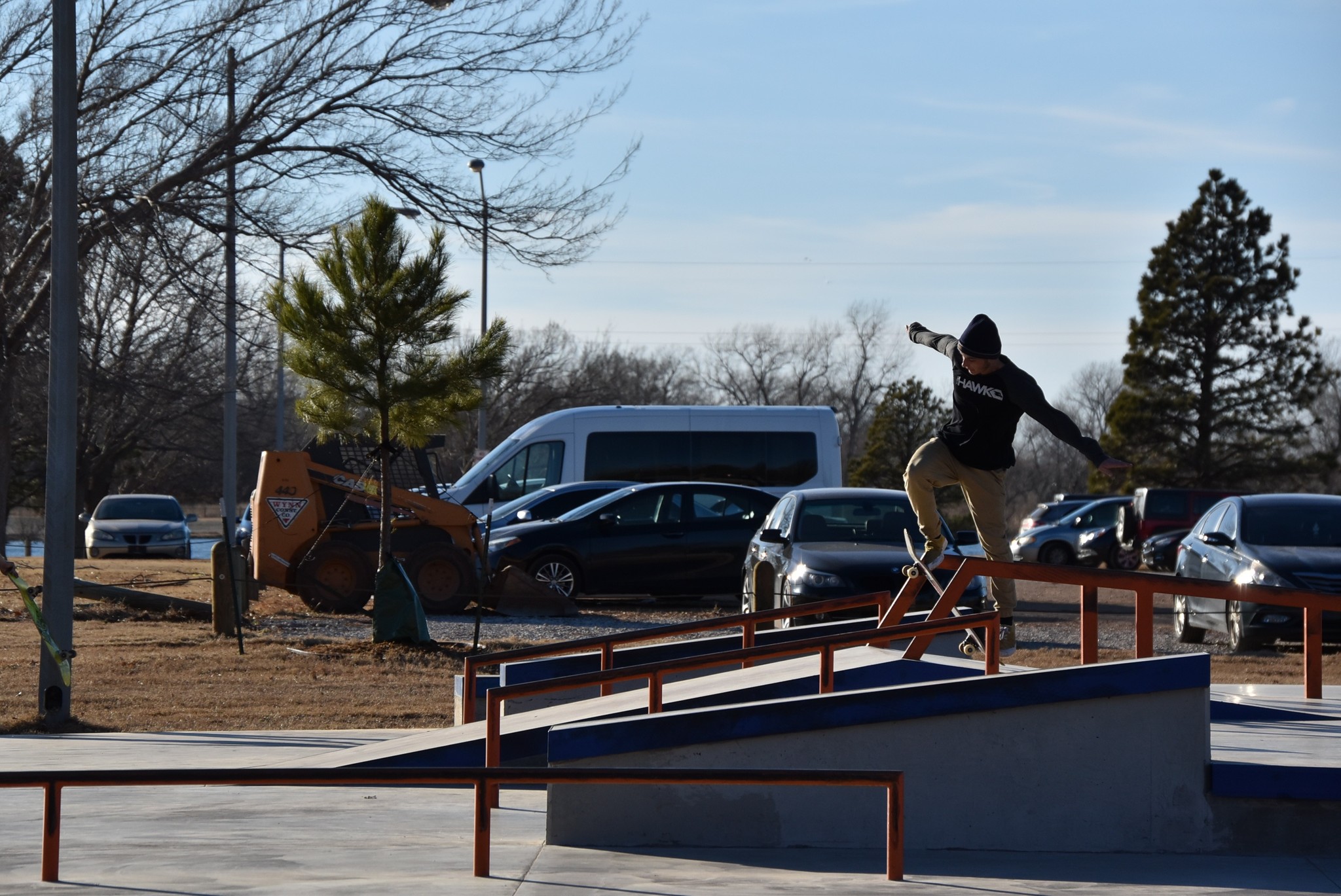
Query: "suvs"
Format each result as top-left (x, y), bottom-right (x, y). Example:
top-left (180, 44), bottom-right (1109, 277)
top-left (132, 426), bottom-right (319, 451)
top-left (1010, 495), bottom-right (1134, 568)
top-left (1116, 486), bottom-right (1241, 551)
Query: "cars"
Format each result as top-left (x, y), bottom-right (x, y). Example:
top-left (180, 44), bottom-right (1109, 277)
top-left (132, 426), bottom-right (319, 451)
top-left (406, 483), bottom-right (454, 498)
top-left (78, 493), bottom-right (198, 559)
top-left (1139, 529), bottom-right (1192, 571)
top-left (234, 503), bottom-right (252, 546)
top-left (472, 480), bottom-right (786, 605)
top-left (1171, 494), bottom-right (1341, 653)
top-left (1077, 524), bottom-right (1144, 571)
top-left (472, 479), bottom-right (647, 535)
top-left (740, 486), bottom-right (989, 630)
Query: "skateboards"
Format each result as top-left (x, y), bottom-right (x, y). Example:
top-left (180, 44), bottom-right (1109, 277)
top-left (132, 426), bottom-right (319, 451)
top-left (900, 528), bottom-right (1008, 668)
top-left (5, 568), bottom-right (77, 687)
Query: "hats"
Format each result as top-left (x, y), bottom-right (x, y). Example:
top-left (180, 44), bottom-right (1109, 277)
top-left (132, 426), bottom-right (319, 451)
top-left (958, 314), bottom-right (1002, 358)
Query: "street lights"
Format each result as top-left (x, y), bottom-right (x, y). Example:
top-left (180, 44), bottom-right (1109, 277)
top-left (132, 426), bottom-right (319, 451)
top-left (469, 155), bottom-right (490, 458)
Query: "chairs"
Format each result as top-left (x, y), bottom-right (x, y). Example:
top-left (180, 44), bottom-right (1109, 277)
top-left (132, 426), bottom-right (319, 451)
top-left (864, 519), bottom-right (884, 532)
top-left (876, 511), bottom-right (909, 535)
top-left (798, 514), bottom-right (828, 541)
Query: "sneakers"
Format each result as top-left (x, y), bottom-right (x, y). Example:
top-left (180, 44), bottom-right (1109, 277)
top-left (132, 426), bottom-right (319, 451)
top-left (918, 534), bottom-right (948, 570)
top-left (999, 619), bottom-right (1017, 656)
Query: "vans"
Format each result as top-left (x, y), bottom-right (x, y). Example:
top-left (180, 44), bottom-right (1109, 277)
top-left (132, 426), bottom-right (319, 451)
top-left (420, 404), bottom-right (843, 517)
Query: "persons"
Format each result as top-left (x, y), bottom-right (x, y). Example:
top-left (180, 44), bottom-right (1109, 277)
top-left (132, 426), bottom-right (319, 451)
top-left (906, 314), bottom-right (1132, 658)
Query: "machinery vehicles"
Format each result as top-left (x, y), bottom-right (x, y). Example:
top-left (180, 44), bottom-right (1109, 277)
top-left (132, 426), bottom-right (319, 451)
top-left (210, 436), bottom-right (582, 635)
